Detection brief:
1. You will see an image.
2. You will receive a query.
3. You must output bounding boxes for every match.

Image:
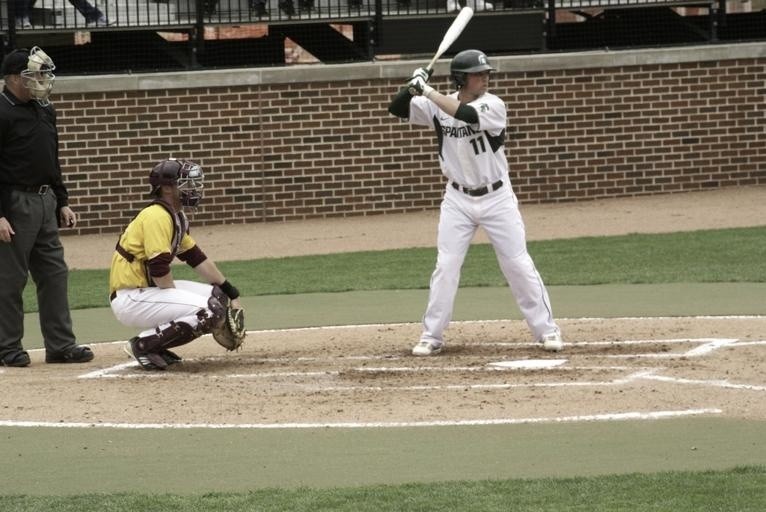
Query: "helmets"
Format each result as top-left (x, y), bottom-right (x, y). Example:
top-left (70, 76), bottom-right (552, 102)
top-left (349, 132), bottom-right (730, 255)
top-left (148, 158), bottom-right (205, 207)
top-left (450, 49), bottom-right (497, 88)
top-left (2, 45), bottom-right (56, 108)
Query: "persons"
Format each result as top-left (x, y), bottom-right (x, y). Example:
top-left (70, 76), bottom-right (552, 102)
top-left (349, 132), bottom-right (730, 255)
top-left (107, 155), bottom-right (240, 371)
top-left (0, 45), bottom-right (93, 367)
top-left (388, 49), bottom-right (564, 357)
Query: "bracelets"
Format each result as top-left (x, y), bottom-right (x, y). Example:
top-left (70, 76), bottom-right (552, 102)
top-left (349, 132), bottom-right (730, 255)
top-left (220, 279), bottom-right (239, 299)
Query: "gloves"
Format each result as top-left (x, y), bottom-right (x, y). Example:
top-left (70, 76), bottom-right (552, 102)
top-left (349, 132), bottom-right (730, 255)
top-left (412, 67), bottom-right (434, 83)
top-left (404, 75), bottom-right (433, 98)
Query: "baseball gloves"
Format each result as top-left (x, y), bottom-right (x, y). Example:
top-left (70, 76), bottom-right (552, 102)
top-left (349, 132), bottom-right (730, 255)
top-left (214, 307), bottom-right (245, 351)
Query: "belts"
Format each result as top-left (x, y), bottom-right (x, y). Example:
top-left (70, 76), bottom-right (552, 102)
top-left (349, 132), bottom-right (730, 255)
top-left (16, 185), bottom-right (52, 194)
top-left (110, 291), bottom-right (116, 301)
top-left (452, 180), bottom-right (502, 197)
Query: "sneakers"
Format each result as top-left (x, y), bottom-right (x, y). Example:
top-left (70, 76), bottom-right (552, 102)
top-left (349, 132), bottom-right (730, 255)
top-left (15, 17), bottom-right (31, 26)
top-left (125, 337), bottom-right (168, 370)
top-left (539, 329), bottom-right (562, 352)
top-left (85, 16), bottom-right (116, 27)
top-left (412, 342), bottom-right (441, 357)
top-left (46, 345), bottom-right (94, 363)
top-left (0, 350), bottom-right (30, 366)
top-left (446, 0), bottom-right (461, 13)
top-left (466, 0), bottom-right (493, 11)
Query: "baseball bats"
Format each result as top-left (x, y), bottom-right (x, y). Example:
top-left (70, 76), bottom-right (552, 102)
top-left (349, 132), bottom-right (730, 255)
top-left (410, 6), bottom-right (474, 96)
